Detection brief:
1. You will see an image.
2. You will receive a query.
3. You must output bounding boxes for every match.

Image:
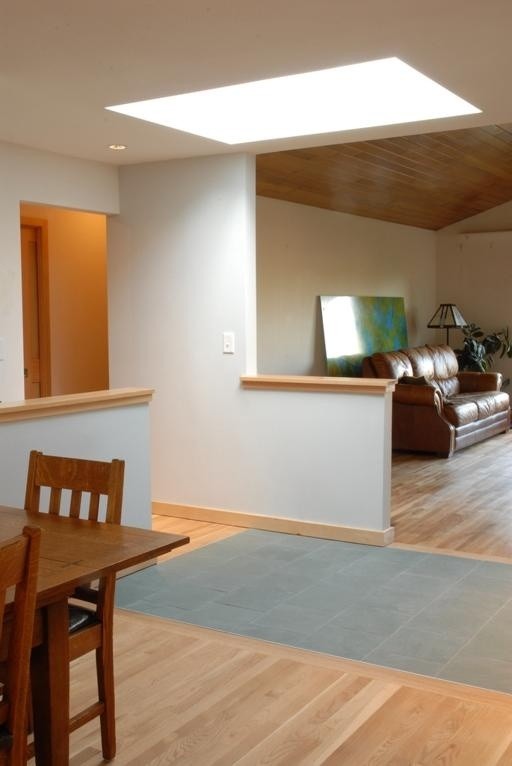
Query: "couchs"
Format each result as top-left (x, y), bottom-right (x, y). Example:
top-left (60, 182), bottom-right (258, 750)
top-left (363, 343), bottom-right (511, 460)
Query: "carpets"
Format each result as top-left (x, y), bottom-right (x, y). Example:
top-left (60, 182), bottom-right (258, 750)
top-left (100, 526), bottom-right (511, 697)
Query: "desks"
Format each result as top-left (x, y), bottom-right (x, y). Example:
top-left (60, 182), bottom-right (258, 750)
top-left (0, 504), bottom-right (189, 766)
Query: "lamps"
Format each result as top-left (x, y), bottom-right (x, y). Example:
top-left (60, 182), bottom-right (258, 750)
top-left (426, 303), bottom-right (469, 346)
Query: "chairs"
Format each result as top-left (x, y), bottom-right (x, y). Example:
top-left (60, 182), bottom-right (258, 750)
top-left (0, 450), bottom-right (124, 762)
top-left (2, 523), bottom-right (43, 766)
